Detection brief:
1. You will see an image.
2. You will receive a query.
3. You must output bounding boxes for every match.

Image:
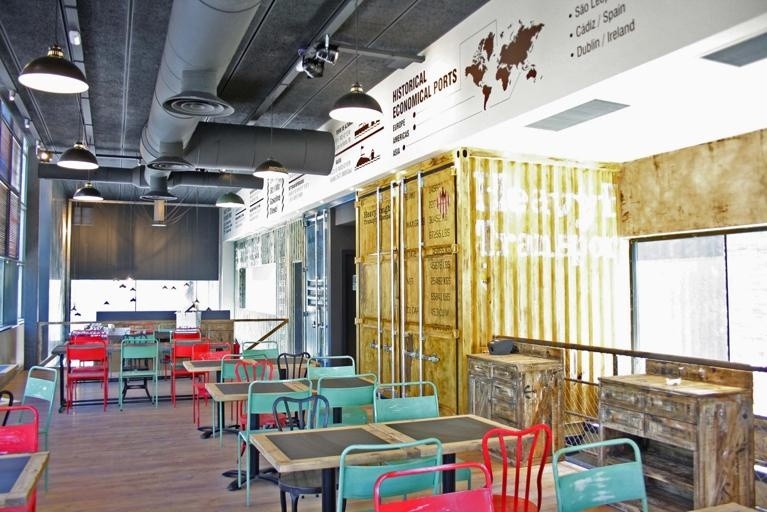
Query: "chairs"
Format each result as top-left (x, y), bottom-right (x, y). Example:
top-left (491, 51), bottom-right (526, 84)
top-left (238, 378), bottom-right (314, 510)
top-left (481, 424), bottom-right (553, 512)
top-left (7, 365), bottom-right (58, 493)
top-left (372, 380), bottom-right (474, 497)
top-left (336, 439), bottom-right (442, 512)
top-left (51, 324), bottom-right (211, 412)
top-left (272, 395), bottom-right (348, 512)
top-left (184, 342), bottom-right (377, 447)
top-left (0, 404), bottom-right (39, 512)
top-left (551, 437), bottom-right (648, 512)
top-left (373, 462), bottom-right (492, 511)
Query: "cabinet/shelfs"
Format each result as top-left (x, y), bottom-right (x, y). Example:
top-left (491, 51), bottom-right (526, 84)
top-left (466, 339), bottom-right (564, 467)
top-left (598, 359), bottom-right (755, 512)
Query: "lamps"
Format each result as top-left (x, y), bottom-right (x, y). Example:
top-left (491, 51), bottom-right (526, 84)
top-left (214, 173), bottom-right (244, 208)
top-left (17, 0), bottom-right (90, 95)
top-left (56, 93), bottom-right (98, 171)
top-left (301, 43), bottom-right (338, 79)
top-left (328, 0), bottom-right (382, 124)
top-left (73, 170), bottom-right (104, 202)
top-left (251, 98), bottom-right (289, 179)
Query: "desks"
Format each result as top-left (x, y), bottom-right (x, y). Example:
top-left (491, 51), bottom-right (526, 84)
top-left (203, 382), bottom-right (306, 491)
top-left (0, 450), bottom-right (50, 506)
top-left (250, 422), bottom-right (407, 511)
top-left (368, 412), bottom-right (535, 495)
